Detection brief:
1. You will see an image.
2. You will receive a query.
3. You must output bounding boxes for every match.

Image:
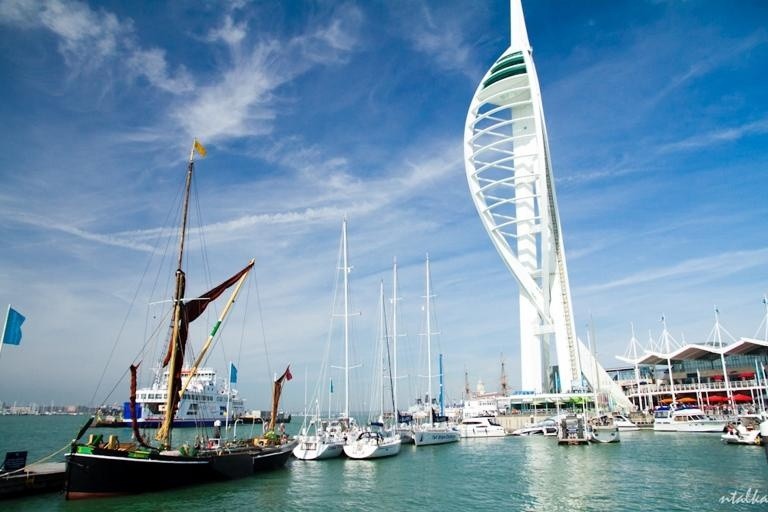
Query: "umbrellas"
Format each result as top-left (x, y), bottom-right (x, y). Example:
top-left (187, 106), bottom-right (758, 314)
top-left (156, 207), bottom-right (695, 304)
top-left (657, 393), bottom-right (768, 404)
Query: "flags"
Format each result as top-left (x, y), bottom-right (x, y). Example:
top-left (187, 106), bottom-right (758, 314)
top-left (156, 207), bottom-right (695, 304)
top-left (195, 142), bottom-right (207, 157)
top-left (230, 364), bottom-right (237, 383)
top-left (2, 307), bottom-right (26, 346)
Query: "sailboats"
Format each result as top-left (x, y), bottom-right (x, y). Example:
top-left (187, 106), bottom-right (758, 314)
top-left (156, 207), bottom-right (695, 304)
top-left (1, 398), bottom-right (58, 416)
top-left (65, 137), bottom-right (299, 501)
top-left (513, 310), bottom-right (621, 445)
top-left (614, 296), bottom-right (768, 451)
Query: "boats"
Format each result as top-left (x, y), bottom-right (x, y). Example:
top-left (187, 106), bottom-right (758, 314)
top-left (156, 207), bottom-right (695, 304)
top-left (90, 341), bottom-right (247, 429)
top-left (241, 410), bottom-right (292, 425)
top-left (448, 415), bottom-right (510, 438)
top-left (0, 451), bottom-right (66, 498)
